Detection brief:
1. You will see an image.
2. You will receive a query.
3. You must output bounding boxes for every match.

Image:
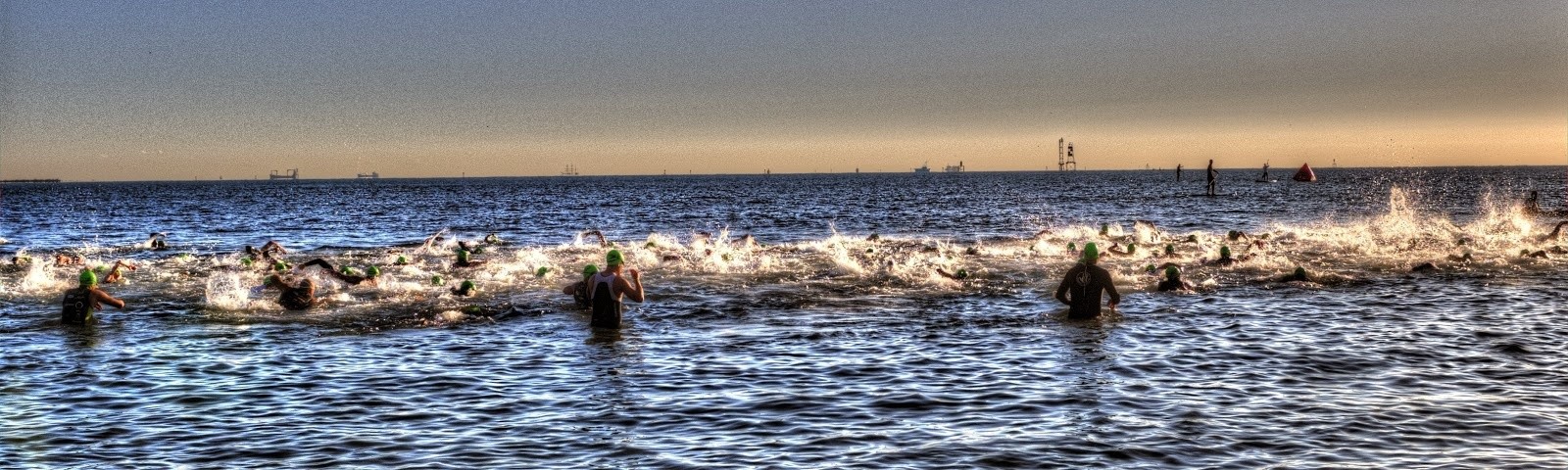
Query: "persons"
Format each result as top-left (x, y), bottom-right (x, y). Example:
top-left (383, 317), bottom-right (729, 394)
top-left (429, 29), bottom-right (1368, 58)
top-left (2, 207), bottom-right (1568, 342)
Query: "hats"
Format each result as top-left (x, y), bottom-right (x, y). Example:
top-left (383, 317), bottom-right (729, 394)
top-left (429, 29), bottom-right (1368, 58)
top-left (1067, 243), bottom-right (1077, 250)
top-left (1083, 242), bottom-right (1099, 260)
top-left (180, 254), bottom-right (186, 258)
top-left (80, 271), bottom-right (97, 285)
top-left (398, 256), bottom-right (407, 263)
top-left (646, 241), bottom-right (656, 247)
top-left (1457, 239), bottom-right (1473, 259)
top-left (433, 239), bottom-right (497, 290)
top-left (340, 266), bottom-right (379, 277)
top-left (607, 241), bottom-right (617, 246)
top-left (241, 258), bottom-right (251, 264)
top-left (867, 233), bottom-right (879, 253)
top-left (1520, 249), bottom-right (1530, 253)
top-left (96, 265), bottom-right (105, 270)
top-left (1295, 267), bottom-right (1306, 275)
top-left (539, 266), bottom-right (548, 274)
top-left (114, 269), bottom-right (121, 275)
top-left (1145, 264), bottom-right (1181, 277)
top-left (957, 269), bottom-right (968, 275)
top-left (607, 250), bottom-right (624, 265)
top-left (583, 265), bottom-right (599, 280)
top-left (1101, 224), bottom-right (1271, 256)
top-left (1554, 246), bottom-right (1561, 249)
top-left (264, 262), bottom-right (292, 286)
top-left (469, 308), bottom-right (481, 313)
